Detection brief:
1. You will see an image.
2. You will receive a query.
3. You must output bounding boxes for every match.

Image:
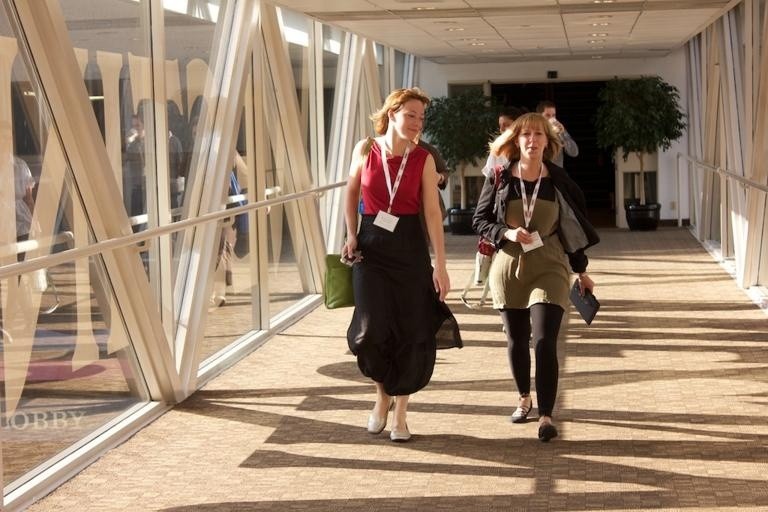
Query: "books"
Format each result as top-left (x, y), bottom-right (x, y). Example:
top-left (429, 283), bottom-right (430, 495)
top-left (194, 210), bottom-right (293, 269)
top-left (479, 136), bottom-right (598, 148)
top-left (569, 278), bottom-right (600, 325)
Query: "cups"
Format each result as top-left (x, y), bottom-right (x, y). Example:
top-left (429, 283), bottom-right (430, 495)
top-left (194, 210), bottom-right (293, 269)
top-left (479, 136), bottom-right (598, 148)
top-left (548, 118), bottom-right (560, 133)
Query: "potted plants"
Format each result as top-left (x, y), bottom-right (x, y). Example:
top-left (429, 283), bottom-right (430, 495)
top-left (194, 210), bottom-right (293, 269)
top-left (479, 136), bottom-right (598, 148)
top-left (422, 90), bottom-right (500, 235)
top-left (584, 72), bottom-right (690, 232)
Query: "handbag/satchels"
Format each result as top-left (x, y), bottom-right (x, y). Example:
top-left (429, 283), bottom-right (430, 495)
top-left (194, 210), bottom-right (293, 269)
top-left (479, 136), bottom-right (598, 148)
top-left (462, 165), bottom-right (505, 311)
top-left (323, 254), bottom-right (355, 309)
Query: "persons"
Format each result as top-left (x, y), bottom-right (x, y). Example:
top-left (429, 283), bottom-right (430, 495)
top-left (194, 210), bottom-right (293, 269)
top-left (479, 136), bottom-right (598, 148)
top-left (415, 138), bottom-right (449, 245)
top-left (0, 115), bottom-right (249, 345)
top-left (481, 106), bottom-right (529, 177)
top-left (536, 101), bottom-right (579, 168)
top-left (343, 87), bottom-right (463, 442)
top-left (471, 112), bottom-right (601, 442)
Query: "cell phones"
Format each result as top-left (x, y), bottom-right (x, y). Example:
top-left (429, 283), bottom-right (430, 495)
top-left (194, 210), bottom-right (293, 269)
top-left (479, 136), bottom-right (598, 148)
top-left (340, 249), bottom-right (362, 266)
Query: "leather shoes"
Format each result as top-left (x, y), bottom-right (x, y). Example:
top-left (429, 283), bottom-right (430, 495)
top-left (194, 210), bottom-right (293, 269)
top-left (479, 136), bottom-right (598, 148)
top-left (367, 395), bottom-right (394, 434)
top-left (511, 399), bottom-right (532, 423)
top-left (389, 421), bottom-right (411, 441)
top-left (538, 423), bottom-right (557, 441)
